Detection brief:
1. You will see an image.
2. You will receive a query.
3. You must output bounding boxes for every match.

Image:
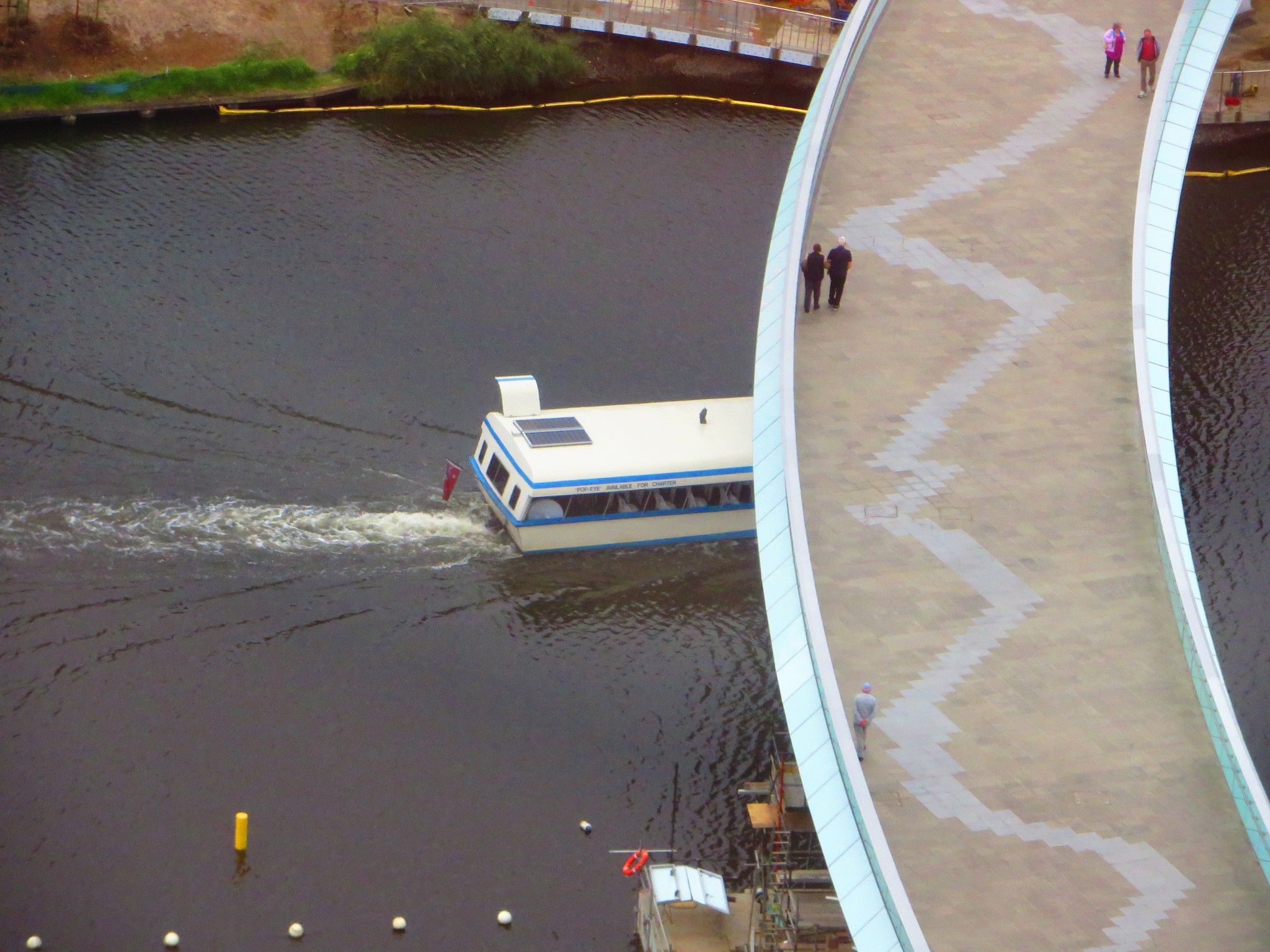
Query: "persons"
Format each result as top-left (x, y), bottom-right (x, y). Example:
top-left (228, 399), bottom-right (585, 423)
top-left (1103, 23), bottom-right (1125, 78)
top-left (853, 681), bottom-right (875, 762)
top-left (801, 237), bottom-right (853, 315)
top-left (1138, 29), bottom-right (1160, 98)
top-left (1231, 69), bottom-right (1241, 96)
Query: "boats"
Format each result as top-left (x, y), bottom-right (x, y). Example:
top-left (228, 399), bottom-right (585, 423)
top-left (604, 760), bottom-right (736, 951)
top-left (473, 374), bottom-right (757, 558)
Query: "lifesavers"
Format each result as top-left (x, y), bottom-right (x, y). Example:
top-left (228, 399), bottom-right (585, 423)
top-left (622, 849), bottom-right (649, 875)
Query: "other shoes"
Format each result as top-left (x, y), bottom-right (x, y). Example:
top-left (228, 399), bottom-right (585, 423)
top-left (859, 757), bottom-right (864, 762)
top-left (813, 305), bottom-right (820, 310)
top-left (1115, 74), bottom-right (1121, 78)
top-left (864, 746), bottom-right (866, 750)
top-left (833, 305), bottom-right (838, 310)
top-left (1105, 73), bottom-right (1109, 78)
top-left (803, 308), bottom-right (810, 313)
top-left (828, 303), bottom-right (833, 307)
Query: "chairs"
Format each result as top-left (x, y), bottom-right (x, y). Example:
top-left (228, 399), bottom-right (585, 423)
top-left (616, 485), bottom-right (742, 515)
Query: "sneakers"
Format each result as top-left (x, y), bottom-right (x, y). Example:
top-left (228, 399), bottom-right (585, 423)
top-left (1138, 91), bottom-right (1147, 98)
top-left (1149, 85), bottom-right (1155, 90)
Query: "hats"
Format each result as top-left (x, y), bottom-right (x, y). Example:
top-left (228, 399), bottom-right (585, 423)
top-left (862, 683), bottom-right (872, 693)
top-left (838, 236), bottom-right (846, 243)
